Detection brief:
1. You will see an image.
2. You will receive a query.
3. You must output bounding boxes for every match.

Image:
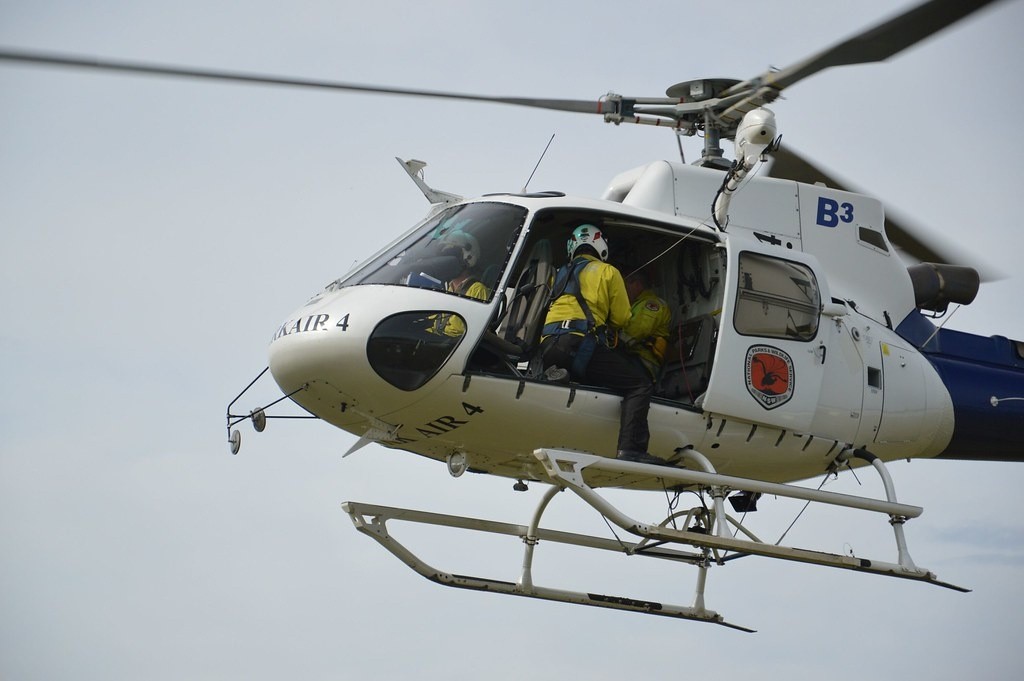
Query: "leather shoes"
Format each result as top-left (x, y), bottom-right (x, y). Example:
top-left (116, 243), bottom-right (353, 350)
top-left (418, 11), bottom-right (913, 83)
top-left (616, 449), bottom-right (669, 466)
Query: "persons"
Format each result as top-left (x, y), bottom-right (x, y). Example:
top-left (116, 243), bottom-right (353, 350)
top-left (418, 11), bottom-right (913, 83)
top-left (604, 262), bottom-right (672, 392)
top-left (544, 224), bottom-right (668, 467)
top-left (421, 232), bottom-right (489, 337)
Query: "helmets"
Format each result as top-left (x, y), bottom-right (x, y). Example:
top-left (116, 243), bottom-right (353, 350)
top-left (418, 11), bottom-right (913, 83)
top-left (437, 230), bottom-right (481, 268)
top-left (566, 224), bottom-right (608, 262)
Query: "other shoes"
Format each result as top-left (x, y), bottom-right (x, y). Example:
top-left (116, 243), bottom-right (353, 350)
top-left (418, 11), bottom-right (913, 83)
top-left (545, 365), bottom-right (567, 380)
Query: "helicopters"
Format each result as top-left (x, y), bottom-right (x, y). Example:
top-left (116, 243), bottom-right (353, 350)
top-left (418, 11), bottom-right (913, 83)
top-left (0, 1), bottom-right (1023, 634)
